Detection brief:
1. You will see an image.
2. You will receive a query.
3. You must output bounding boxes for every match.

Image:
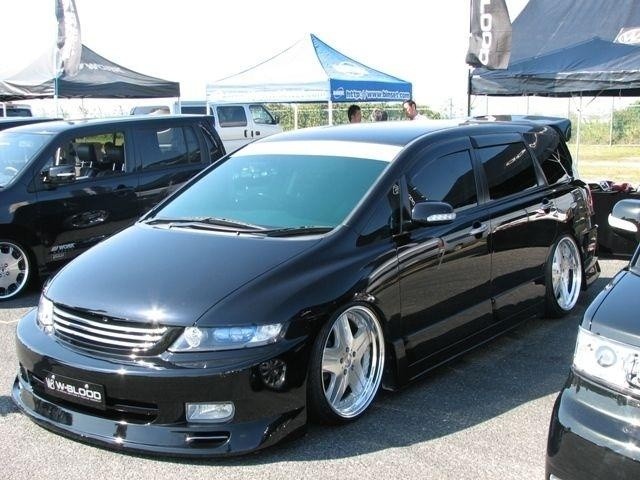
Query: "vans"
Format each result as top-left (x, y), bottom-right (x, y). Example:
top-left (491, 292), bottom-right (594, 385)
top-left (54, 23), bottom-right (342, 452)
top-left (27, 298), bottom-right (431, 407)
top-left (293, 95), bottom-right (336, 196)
top-left (129, 103), bottom-right (285, 155)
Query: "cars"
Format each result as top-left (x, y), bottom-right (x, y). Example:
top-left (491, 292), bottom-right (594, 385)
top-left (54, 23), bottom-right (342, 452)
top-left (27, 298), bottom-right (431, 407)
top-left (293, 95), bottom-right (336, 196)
top-left (8, 113), bottom-right (603, 460)
top-left (542, 197), bottom-right (640, 480)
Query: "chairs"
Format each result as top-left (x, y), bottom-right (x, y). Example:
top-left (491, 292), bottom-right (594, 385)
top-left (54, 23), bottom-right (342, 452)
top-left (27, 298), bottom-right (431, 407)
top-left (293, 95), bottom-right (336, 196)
top-left (75, 142), bottom-right (125, 179)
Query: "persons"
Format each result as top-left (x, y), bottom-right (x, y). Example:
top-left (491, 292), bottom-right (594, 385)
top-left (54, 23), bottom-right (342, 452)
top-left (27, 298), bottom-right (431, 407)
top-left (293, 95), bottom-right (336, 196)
top-left (347, 104), bottom-right (363, 123)
top-left (368, 107), bottom-right (388, 121)
top-left (402, 99), bottom-right (429, 120)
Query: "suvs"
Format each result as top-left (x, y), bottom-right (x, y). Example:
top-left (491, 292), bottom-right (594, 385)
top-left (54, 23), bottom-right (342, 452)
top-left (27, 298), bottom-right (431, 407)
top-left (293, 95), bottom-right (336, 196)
top-left (0, 115), bottom-right (231, 303)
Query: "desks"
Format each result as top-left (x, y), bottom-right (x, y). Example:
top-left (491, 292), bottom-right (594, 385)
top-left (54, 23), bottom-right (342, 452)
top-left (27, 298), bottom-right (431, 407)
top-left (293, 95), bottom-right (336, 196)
top-left (589, 190), bottom-right (640, 260)
